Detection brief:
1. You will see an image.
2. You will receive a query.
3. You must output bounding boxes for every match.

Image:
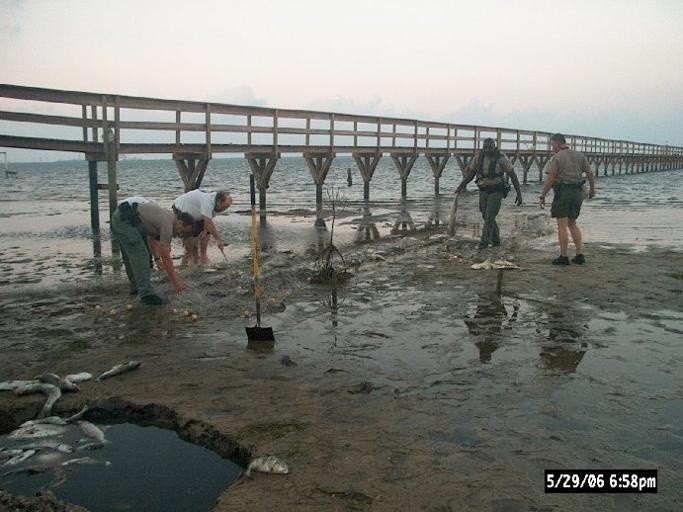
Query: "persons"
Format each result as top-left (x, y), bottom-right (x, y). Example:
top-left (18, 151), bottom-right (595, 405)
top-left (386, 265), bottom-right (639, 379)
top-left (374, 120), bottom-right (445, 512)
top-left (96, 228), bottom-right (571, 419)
top-left (464, 293), bottom-right (520, 364)
top-left (110, 196), bottom-right (196, 305)
top-left (536, 313), bottom-right (589, 375)
top-left (172, 189), bottom-right (233, 265)
top-left (454, 138), bottom-right (522, 251)
top-left (538, 133), bottom-right (595, 266)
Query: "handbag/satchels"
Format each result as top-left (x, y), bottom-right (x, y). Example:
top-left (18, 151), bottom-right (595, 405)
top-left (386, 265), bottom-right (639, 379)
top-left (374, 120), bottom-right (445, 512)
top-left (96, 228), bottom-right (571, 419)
top-left (118, 201), bottom-right (140, 226)
top-left (503, 182), bottom-right (511, 199)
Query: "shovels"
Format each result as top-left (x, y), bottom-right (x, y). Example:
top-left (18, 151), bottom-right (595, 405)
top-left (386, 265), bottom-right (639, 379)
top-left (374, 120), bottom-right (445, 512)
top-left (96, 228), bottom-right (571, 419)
top-left (244, 174), bottom-right (275, 342)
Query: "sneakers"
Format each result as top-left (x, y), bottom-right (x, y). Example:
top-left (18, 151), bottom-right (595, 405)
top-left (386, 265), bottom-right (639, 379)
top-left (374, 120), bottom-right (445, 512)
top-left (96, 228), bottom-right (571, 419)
top-left (552, 253), bottom-right (585, 265)
top-left (128, 291), bottom-right (168, 305)
top-left (478, 240), bottom-right (500, 249)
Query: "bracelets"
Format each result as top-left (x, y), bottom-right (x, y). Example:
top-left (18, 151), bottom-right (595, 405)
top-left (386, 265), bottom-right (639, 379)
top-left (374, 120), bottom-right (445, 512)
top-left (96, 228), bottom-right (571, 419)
top-left (154, 258), bottom-right (160, 261)
top-left (540, 197), bottom-right (545, 200)
